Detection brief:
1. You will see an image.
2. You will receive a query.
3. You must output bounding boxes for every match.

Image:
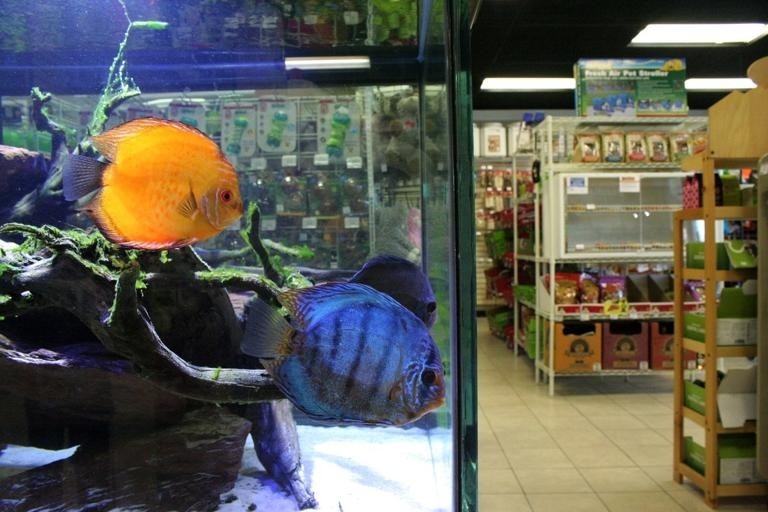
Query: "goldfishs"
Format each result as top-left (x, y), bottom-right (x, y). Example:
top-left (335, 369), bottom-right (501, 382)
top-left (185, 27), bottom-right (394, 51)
top-left (241, 279), bottom-right (446, 427)
top-left (62, 116), bottom-right (247, 250)
top-left (340, 255), bottom-right (437, 330)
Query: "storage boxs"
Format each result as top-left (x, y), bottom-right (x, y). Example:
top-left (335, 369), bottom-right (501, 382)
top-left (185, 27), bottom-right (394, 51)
top-left (651, 321), bottom-right (675, 369)
top-left (574, 56), bottom-right (689, 117)
top-left (544, 319), bottom-right (603, 373)
top-left (604, 324), bottom-right (648, 369)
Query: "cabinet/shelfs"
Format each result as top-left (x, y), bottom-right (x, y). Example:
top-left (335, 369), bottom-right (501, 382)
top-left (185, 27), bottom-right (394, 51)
top-left (532, 117), bottom-right (708, 398)
top-left (0, 87), bottom-right (375, 287)
top-left (670, 157), bottom-right (768, 508)
top-left (512, 156), bottom-right (532, 368)
top-left (475, 155), bottom-right (512, 313)
